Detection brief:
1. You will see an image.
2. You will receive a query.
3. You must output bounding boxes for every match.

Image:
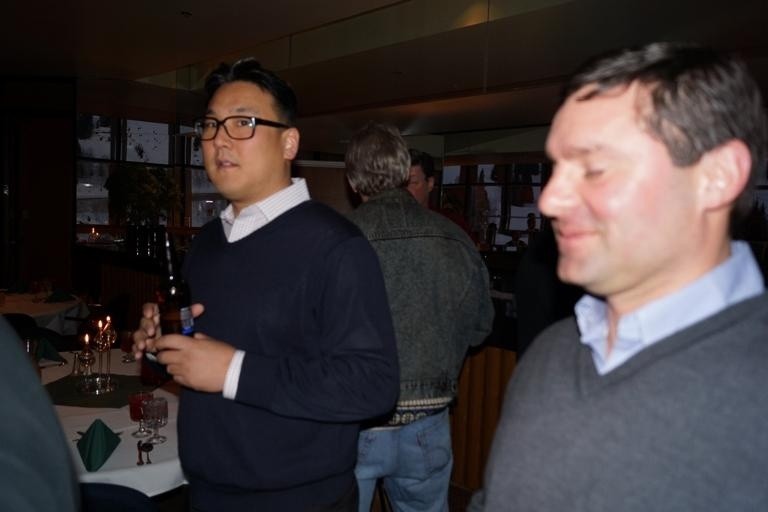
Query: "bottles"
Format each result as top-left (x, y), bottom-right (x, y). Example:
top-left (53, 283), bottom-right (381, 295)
top-left (156, 230), bottom-right (195, 341)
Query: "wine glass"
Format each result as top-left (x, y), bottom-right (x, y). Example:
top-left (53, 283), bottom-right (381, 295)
top-left (119, 331), bottom-right (138, 365)
top-left (130, 387), bottom-right (169, 446)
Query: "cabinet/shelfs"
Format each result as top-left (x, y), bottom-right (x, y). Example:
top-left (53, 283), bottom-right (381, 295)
top-left (79, 116), bottom-right (179, 233)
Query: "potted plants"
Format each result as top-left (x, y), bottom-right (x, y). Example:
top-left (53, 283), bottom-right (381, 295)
top-left (104, 163), bottom-right (176, 251)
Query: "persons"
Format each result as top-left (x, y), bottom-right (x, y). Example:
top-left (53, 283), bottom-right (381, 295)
top-left (0, 310), bottom-right (88, 511)
top-left (462, 35), bottom-right (766, 511)
top-left (126, 54), bottom-right (406, 512)
top-left (405, 147), bottom-right (435, 207)
top-left (336, 115), bottom-right (496, 511)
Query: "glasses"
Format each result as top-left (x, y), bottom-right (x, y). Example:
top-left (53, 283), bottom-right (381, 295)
top-left (195, 116), bottom-right (290, 141)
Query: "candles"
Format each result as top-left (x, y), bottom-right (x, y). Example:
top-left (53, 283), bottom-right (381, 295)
top-left (97, 318), bottom-right (108, 345)
top-left (103, 316), bottom-right (116, 336)
top-left (82, 335), bottom-right (90, 347)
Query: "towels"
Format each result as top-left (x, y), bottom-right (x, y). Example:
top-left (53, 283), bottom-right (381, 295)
top-left (42, 290), bottom-right (75, 301)
top-left (76, 416), bottom-right (119, 472)
